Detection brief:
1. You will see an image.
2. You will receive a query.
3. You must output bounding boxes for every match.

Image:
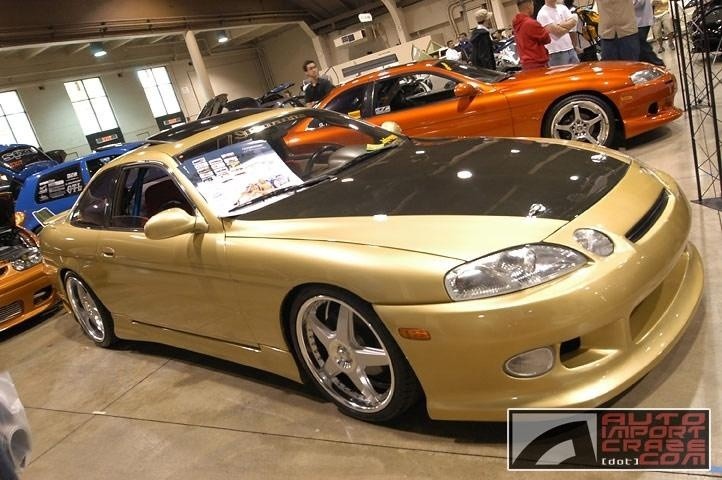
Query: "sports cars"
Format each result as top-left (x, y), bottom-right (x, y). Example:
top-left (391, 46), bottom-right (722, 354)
top-left (283, 59), bottom-right (681, 149)
top-left (43, 106), bottom-right (705, 427)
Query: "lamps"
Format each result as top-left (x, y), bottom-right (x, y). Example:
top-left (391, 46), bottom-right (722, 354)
top-left (90, 42), bottom-right (107, 57)
top-left (216, 30), bottom-right (228, 43)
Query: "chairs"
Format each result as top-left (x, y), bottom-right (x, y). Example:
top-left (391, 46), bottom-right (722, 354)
top-left (144, 180), bottom-right (181, 218)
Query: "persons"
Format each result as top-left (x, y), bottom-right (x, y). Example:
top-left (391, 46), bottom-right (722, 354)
top-left (594, 0), bottom-right (641, 61)
top-left (301, 58), bottom-right (335, 106)
top-left (537, 0), bottom-right (583, 67)
top-left (442, 25), bottom-right (516, 62)
top-left (284, 90), bottom-right (293, 99)
top-left (563, 0), bottom-right (599, 63)
top-left (466, 7), bottom-right (496, 70)
top-left (632, 0), bottom-right (667, 68)
top-left (648, 0), bottom-right (675, 55)
top-left (511, 0), bottom-right (557, 71)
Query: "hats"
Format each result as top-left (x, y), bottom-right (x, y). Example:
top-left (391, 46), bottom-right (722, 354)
top-left (474, 8), bottom-right (493, 24)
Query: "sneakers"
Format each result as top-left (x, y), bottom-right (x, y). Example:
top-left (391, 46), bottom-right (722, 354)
top-left (658, 47), bottom-right (665, 54)
top-left (669, 42), bottom-right (675, 50)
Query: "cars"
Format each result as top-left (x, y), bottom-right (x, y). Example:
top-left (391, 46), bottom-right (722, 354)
top-left (197, 94), bottom-right (228, 119)
top-left (0, 142), bottom-right (148, 238)
top-left (1, 192), bottom-right (62, 335)
top-left (690, 0), bottom-right (722, 56)
top-left (0, 404), bottom-right (32, 479)
top-left (262, 98), bottom-right (303, 108)
